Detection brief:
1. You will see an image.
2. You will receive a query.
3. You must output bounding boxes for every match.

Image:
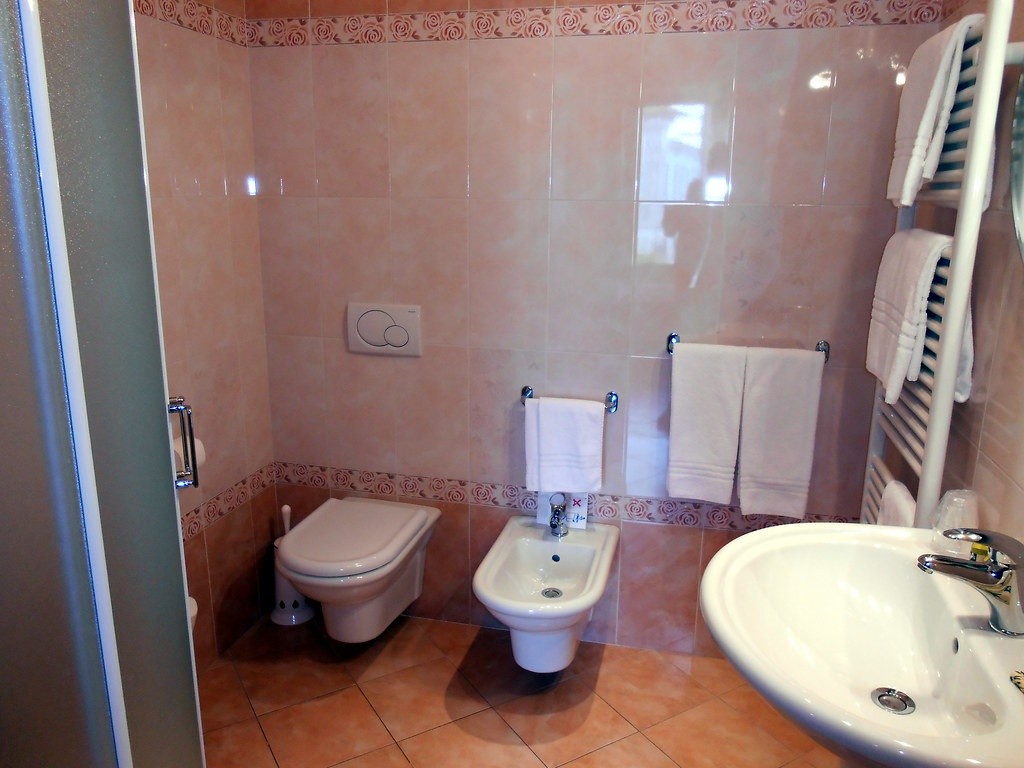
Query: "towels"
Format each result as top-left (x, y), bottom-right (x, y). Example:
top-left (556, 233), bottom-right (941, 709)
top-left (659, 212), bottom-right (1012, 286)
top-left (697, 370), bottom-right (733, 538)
top-left (885, 13), bottom-right (998, 213)
top-left (876, 480), bottom-right (917, 527)
top-left (866, 225), bottom-right (976, 404)
top-left (736, 347), bottom-right (825, 518)
top-left (525, 397), bottom-right (605, 492)
top-left (666, 343), bottom-right (747, 505)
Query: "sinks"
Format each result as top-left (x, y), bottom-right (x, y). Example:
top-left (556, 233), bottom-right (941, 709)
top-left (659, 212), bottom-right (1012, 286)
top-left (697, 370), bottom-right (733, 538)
top-left (701, 522), bottom-right (1024, 768)
top-left (473, 517), bottom-right (619, 673)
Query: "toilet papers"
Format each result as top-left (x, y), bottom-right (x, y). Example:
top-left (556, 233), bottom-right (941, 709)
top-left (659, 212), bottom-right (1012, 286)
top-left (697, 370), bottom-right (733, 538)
top-left (173, 436), bottom-right (206, 474)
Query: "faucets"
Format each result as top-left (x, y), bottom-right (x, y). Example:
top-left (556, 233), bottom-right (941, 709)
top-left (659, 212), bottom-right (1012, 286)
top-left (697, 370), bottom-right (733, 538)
top-left (915, 523), bottom-right (1024, 638)
top-left (549, 493), bottom-right (569, 538)
top-left (917, 527), bottom-right (1023, 639)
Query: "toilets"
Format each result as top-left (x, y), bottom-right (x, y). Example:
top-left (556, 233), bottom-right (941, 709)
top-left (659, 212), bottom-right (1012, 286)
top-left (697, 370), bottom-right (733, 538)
top-left (277, 499), bottom-right (440, 642)
